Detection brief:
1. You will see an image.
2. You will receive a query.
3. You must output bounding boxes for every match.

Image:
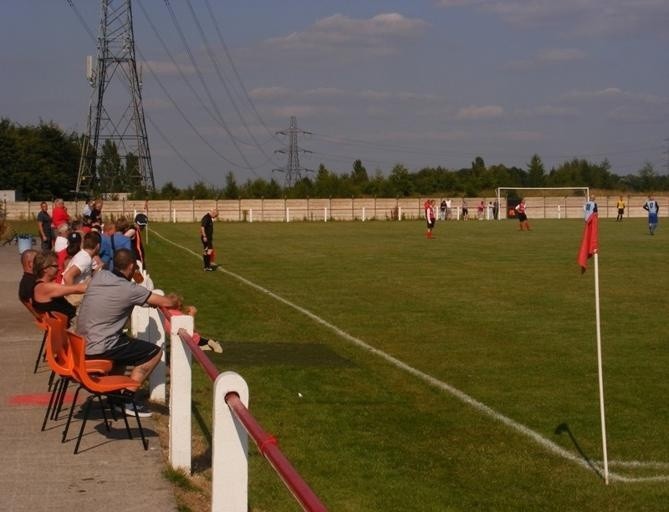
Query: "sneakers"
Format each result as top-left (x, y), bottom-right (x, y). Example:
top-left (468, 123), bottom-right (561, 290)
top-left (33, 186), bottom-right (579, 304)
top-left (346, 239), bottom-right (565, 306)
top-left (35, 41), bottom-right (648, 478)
top-left (104, 398), bottom-right (114, 412)
top-left (204, 266), bottom-right (215, 271)
top-left (114, 401), bottom-right (153, 418)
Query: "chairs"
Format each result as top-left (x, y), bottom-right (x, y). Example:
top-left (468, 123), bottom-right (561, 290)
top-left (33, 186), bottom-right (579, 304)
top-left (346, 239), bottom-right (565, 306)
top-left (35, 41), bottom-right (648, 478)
top-left (25, 298), bottom-right (148, 454)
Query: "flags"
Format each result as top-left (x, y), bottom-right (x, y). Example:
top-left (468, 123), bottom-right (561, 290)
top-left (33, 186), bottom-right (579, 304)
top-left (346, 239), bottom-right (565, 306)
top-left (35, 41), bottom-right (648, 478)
top-left (577, 211), bottom-right (600, 280)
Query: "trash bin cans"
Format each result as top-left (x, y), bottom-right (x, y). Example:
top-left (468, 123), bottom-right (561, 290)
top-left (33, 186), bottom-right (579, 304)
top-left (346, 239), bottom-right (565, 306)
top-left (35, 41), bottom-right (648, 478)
top-left (16, 234), bottom-right (32, 254)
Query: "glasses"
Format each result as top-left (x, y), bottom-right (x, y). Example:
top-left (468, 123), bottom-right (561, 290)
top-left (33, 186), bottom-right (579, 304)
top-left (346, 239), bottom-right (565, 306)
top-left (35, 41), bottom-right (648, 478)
top-left (40, 265), bottom-right (58, 271)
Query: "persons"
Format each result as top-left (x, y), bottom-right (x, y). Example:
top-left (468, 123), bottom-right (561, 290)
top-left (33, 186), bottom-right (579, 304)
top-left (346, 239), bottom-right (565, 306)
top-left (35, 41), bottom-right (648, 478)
top-left (477, 201), bottom-right (484, 221)
top-left (515, 200), bottom-right (533, 231)
top-left (440, 200), bottom-right (447, 221)
top-left (462, 201), bottom-right (468, 221)
top-left (424, 200), bottom-right (435, 239)
top-left (616, 196), bottom-right (625, 222)
top-left (20, 197), bottom-right (197, 419)
top-left (583, 194), bottom-right (598, 228)
top-left (200, 209), bottom-right (219, 271)
top-left (642, 194), bottom-right (659, 235)
top-left (487, 202), bottom-right (494, 220)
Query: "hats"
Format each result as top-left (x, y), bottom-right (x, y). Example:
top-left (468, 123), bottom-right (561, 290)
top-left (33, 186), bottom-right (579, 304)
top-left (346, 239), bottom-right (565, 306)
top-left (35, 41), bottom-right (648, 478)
top-left (136, 214), bottom-right (148, 226)
top-left (67, 232), bottom-right (80, 241)
